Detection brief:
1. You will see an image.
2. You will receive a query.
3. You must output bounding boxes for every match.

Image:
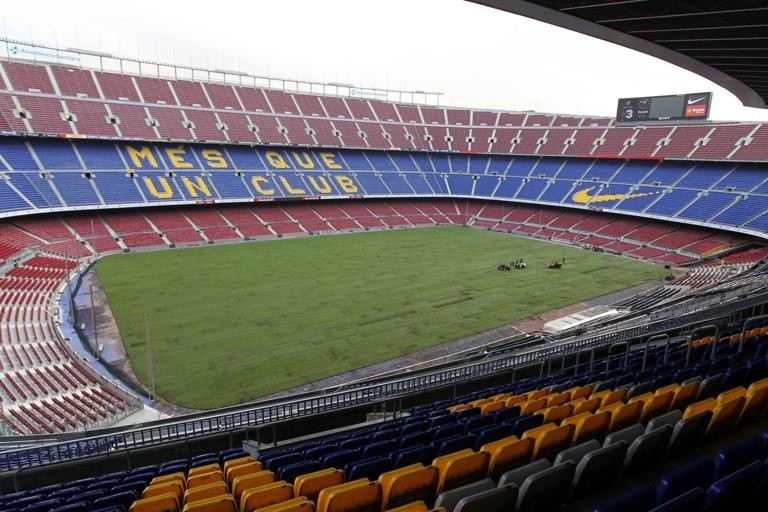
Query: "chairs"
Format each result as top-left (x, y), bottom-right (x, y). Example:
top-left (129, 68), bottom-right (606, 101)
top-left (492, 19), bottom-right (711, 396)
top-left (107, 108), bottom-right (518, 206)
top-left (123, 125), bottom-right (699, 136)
top-left (1, 61), bottom-right (768, 512)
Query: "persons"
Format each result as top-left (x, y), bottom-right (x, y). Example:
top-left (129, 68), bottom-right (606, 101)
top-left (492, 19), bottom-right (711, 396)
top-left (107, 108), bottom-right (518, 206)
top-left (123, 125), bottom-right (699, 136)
top-left (510, 255), bottom-right (565, 270)
